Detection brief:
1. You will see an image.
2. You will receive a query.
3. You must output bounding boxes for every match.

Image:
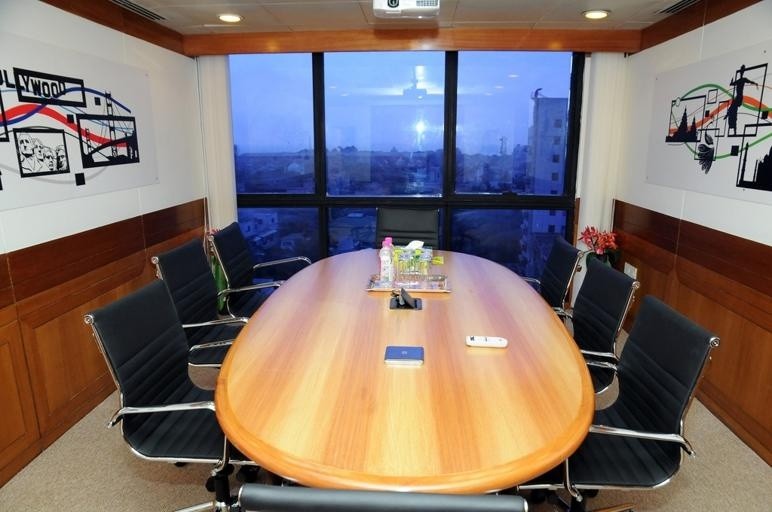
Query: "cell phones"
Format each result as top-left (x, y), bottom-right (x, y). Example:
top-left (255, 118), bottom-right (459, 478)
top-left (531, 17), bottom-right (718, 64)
top-left (466, 335), bottom-right (508, 349)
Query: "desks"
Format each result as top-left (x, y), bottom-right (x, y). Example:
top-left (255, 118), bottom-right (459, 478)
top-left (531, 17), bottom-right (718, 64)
top-left (213, 246), bottom-right (598, 496)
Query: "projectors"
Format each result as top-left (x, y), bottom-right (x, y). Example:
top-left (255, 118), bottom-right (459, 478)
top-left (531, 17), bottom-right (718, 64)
top-left (372, 0), bottom-right (440, 19)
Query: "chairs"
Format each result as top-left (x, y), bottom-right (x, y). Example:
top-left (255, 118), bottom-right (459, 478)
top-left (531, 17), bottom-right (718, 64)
top-left (83, 278), bottom-right (260, 491)
top-left (150, 237), bottom-right (285, 369)
top-left (555, 255), bottom-right (642, 396)
top-left (374, 205), bottom-right (442, 250)
top-left (206, 221), bottom-right (312, 317)
top-left (514, 292), bottom-right (722, 512)
top-left (522, 234), bottom-right (587, 313)
top-left (238, 483), bottom-right (529, 512)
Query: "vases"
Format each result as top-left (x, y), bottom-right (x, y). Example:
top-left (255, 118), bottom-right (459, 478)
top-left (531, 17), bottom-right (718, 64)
top-left (586, 252), bottom-right (608, 268)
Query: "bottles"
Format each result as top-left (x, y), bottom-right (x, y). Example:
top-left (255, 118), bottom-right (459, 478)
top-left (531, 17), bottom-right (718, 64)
top-left (378, 241), bottom-right (394, 287)
top-left (386, 238), bottom-right (394, 255)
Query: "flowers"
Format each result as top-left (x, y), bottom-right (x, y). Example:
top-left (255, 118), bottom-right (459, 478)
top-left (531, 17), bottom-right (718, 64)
top-left (577, 224), bottom-right (618, 254)
